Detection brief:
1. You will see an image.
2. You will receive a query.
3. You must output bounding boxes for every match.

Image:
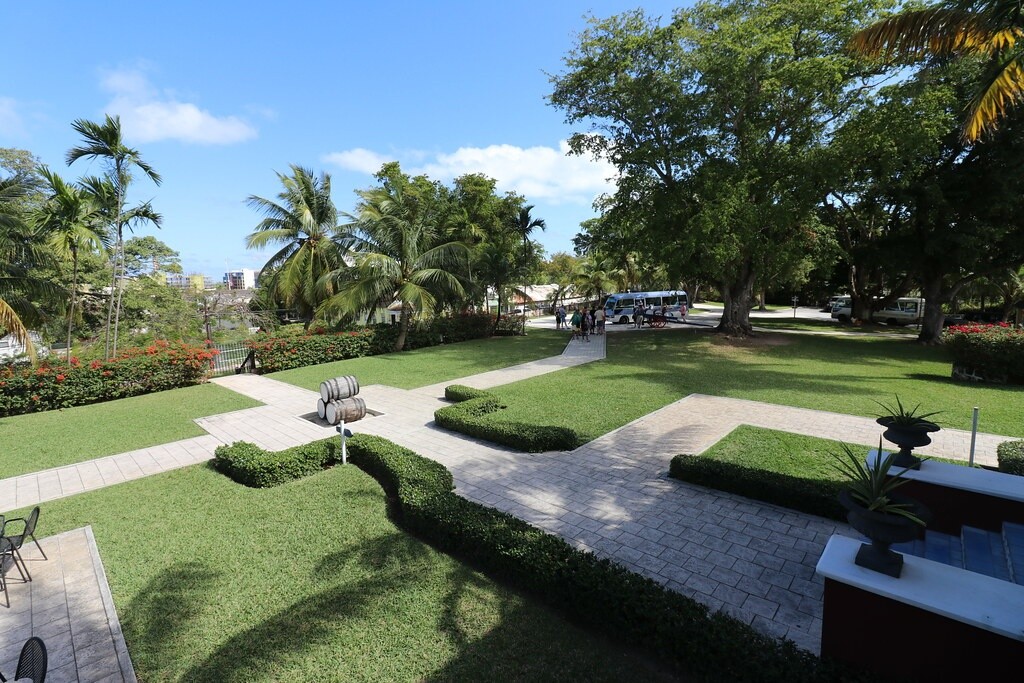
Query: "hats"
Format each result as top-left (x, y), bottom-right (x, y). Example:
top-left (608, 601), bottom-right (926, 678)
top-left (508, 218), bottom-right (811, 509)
top-left (638, 301), bottom-right (643, 306)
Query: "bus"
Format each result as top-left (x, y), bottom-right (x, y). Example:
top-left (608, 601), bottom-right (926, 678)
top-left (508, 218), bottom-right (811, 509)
top-left (603, 290), bottom-right (689, 328)
top-left (830, 295), bottom-right (925, 327)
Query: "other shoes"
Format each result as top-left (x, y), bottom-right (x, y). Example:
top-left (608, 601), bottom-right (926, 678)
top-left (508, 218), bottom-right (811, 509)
top-left (567, 327), bottom-right (571, 330)
top-left (557, 327), bottom-right (560, 330)
top-left (582, 339), bottom-right (591, 343)
top-left (571, 331), bottom-right (604, 339)
top-left (634, 326), bottom-right (636, 328)
top-left (638, 326), bottom-right (641, 329)
top-left (684, 321), bottom-right (686, 323)
top-left (562, 327), bottom-right (564, 330)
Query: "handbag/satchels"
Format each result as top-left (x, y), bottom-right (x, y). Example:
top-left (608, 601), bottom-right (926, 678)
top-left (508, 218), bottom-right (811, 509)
top-left (562, 314), bottom-right (566, 317)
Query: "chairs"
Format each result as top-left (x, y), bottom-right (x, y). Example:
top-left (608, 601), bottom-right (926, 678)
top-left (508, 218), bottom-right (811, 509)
top-left (14, 637), bottom-right (47, 683)
top-left (0, 506), bottom-right (48, 608)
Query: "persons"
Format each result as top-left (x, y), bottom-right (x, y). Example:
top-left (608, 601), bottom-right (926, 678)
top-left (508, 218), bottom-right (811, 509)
top-left (555, 305), bottom-right (569, 330)
top-left (568, 305), bottom-right (606, 342)
top-left (680, 303), bottom-right (687, 323)
top-left (631, 301), bottom-right (668, 329)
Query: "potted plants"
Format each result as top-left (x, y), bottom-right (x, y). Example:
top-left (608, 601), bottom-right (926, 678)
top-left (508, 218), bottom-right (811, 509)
top-left (825, 433), bottom-right (930, 578)
top-left (865, 393), bottom-right (946, 470)
